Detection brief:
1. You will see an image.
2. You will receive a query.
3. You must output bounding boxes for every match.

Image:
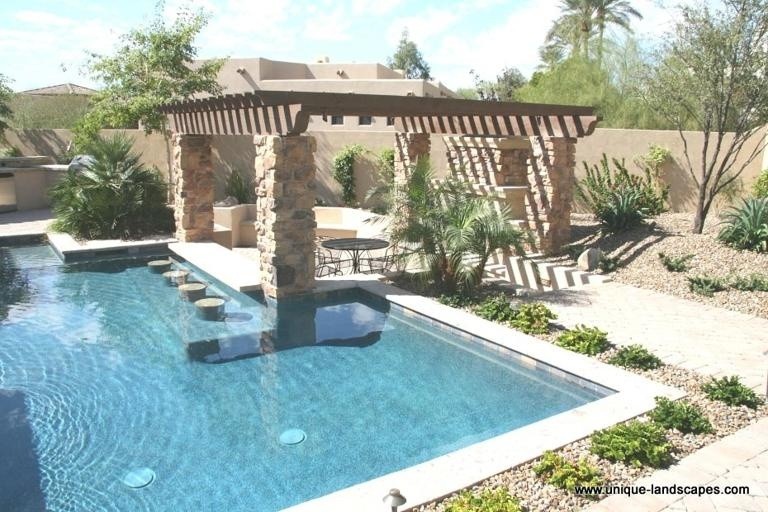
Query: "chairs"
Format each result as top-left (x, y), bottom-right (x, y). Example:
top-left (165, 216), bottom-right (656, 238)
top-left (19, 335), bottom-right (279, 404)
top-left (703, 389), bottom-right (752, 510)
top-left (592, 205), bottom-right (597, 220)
top-left (315, 237), bottom-right (401, 278)
